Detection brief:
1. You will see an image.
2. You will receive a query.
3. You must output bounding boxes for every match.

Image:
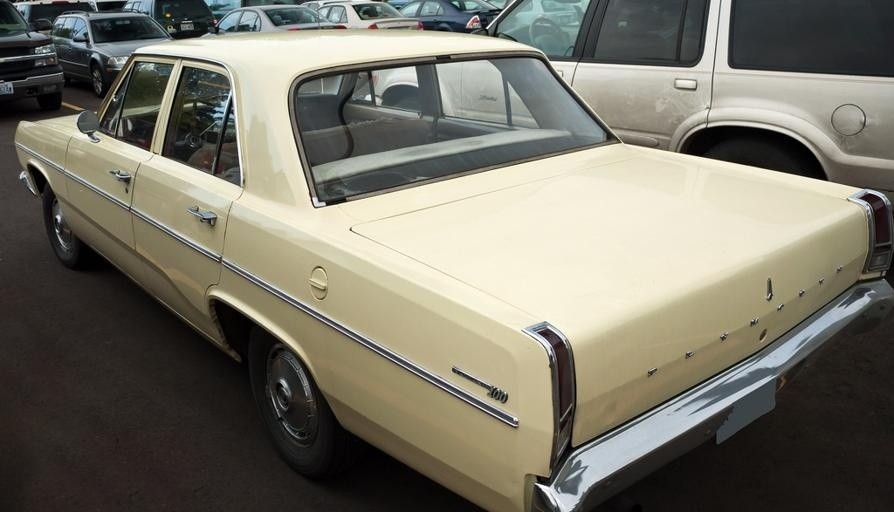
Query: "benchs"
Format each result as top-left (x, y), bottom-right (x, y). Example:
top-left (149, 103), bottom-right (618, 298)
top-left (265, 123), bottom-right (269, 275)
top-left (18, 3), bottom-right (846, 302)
top-left (187, 114), bottom-right (434, 178)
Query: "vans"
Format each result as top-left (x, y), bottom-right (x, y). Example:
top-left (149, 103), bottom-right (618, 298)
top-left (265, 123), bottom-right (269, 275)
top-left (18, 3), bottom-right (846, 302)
top-left (363, 1), bottom-right (893, 226)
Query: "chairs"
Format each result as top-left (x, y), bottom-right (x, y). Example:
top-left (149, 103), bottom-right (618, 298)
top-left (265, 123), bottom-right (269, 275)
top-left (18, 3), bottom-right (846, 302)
top-left (678, 21), bottom-right (701, 63)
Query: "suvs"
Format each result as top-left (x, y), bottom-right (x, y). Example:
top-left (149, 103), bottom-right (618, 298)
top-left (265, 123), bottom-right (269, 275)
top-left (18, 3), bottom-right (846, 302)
top-left (122, 0), bottom-right (219, 39)
top-left (13, 0), bottom-right (96, 24)
top-left (51, 10), bottom-right (179, 99)
top-left (1, 0), bottom-right (67, 112)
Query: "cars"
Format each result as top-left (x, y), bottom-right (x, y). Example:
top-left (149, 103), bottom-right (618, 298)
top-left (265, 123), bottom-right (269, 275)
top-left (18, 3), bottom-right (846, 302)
top-left (12, 28), bottom-right (893, 512)
top-left (207, 1), bottom-right (581, 33)
